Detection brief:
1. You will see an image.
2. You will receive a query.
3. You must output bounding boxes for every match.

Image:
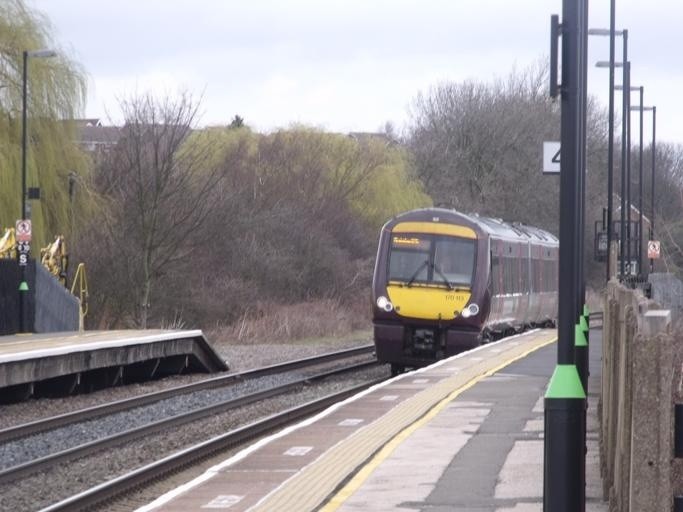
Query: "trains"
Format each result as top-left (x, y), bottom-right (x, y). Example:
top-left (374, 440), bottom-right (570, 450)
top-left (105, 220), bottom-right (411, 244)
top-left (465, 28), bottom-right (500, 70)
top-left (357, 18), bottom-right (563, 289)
top-left (367, 206), bottom-right (560, 379)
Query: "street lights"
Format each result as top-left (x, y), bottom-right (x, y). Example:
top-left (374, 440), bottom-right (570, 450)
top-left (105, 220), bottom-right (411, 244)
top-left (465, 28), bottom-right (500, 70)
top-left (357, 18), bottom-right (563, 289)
top-left (21, 49), bottom-right (57, 334)
top-left (588, 26), bottom-right (653, 286)
